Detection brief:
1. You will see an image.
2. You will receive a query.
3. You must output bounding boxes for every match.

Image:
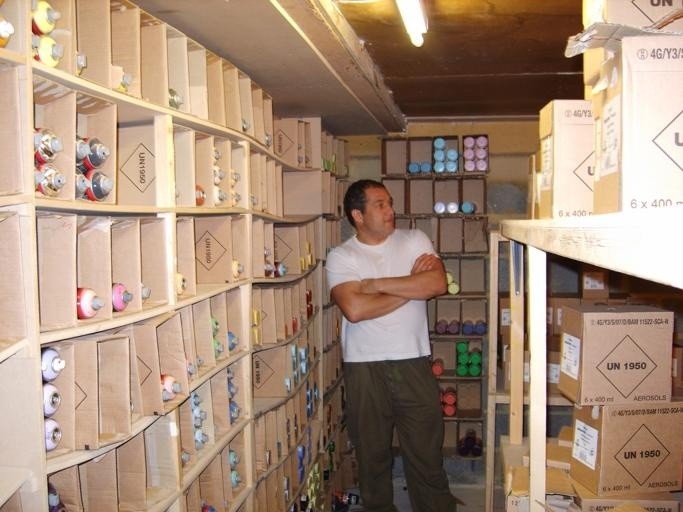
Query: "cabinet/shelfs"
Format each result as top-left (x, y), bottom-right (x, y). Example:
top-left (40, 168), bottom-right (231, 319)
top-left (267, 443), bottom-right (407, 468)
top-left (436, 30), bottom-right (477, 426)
top-left (477, 206), bottom-right (682, 510)
top-left (376, 134), bottom-right (493, 461)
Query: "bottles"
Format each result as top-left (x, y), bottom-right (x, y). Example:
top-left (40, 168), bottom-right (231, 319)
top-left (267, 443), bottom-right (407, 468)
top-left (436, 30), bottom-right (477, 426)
top-left (431, 319), bottom-right (487, 456)
top-left (75, 51), bottom-right (85, 75)
top-left (117, 67), bottom-right (130, 91)
top-left (47, 492), bottom-right (69, 511)
top-left (333, 489), bottom-right (361, 507)
top-left (0, 2), bottom-right (63, 67)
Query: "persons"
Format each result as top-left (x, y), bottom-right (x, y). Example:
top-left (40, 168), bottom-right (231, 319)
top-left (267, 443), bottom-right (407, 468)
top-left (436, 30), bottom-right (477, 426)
top-left (324, 178), bottom-right (460, 512)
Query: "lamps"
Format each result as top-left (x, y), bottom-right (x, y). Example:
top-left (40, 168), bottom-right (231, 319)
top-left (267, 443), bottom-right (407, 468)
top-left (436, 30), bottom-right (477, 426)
top-left (394, 0), bottom-right (431, 49)
